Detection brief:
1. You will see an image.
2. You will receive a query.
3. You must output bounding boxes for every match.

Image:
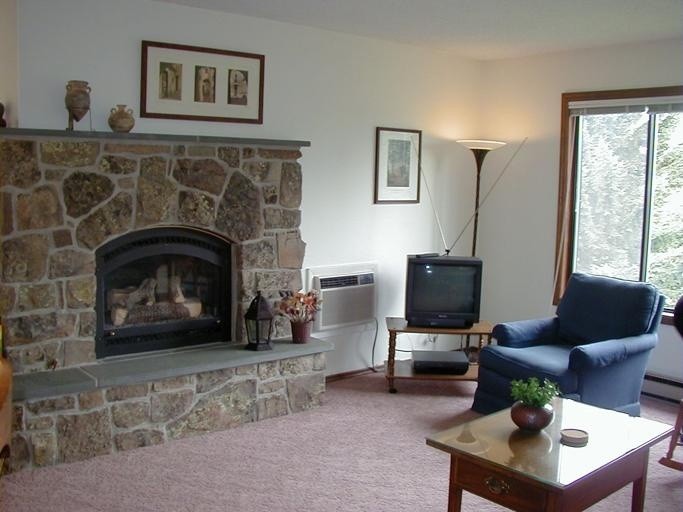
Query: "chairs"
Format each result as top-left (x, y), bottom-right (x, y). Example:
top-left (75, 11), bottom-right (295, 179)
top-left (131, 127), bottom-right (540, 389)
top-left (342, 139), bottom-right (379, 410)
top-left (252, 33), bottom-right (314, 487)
top-left (472, 272), bottom-right (667, 417)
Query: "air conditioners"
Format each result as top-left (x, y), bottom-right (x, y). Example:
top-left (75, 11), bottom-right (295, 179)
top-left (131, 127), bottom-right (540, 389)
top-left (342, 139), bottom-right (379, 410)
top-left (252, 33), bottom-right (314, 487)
top-left (306, 261), bottom-right (376, 331)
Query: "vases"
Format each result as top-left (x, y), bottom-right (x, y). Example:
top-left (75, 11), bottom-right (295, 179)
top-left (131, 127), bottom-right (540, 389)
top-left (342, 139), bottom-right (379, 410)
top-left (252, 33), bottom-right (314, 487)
top-left (291, 320), bottom-right (313, 343)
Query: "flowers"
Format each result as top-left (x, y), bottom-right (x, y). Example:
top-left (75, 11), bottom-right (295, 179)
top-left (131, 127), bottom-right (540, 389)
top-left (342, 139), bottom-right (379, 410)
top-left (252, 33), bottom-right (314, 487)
top-left (272, 287), bottom-right (323, 323)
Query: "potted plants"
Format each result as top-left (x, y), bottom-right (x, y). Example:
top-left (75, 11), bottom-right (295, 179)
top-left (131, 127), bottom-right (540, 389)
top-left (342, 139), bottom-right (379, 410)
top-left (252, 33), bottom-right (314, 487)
top-left (508, 377), bottom-right (562, 434)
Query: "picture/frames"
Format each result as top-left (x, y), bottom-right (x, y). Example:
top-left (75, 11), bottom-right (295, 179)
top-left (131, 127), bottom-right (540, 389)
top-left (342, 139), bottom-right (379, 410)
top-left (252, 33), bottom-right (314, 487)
top-left (140, 40), bottom-right (264, 124)
top-left (375, 127), bottom-right (421, 203)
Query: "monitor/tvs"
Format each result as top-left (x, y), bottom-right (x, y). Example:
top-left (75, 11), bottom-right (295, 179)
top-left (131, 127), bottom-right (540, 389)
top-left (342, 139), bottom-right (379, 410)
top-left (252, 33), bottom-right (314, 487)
top-left (405, 255), bottom-right (483, 329)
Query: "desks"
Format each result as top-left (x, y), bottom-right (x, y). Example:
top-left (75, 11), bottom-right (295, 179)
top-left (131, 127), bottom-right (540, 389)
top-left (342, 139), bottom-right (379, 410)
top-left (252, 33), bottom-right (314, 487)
top-left (385, 317), bottom-right (493, 389)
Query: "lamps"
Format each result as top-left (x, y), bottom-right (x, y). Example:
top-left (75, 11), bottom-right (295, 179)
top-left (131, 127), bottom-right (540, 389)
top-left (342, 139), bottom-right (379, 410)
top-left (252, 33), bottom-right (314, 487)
top-left (457, 140), bottom-right (507, 258)
top-left (243, 291), bottom-right (274, 350)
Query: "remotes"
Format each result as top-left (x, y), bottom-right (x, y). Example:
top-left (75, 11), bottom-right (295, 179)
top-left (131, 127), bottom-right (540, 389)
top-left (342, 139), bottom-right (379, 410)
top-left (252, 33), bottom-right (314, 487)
top-left (417, 253), bottom-right (438, 257)
